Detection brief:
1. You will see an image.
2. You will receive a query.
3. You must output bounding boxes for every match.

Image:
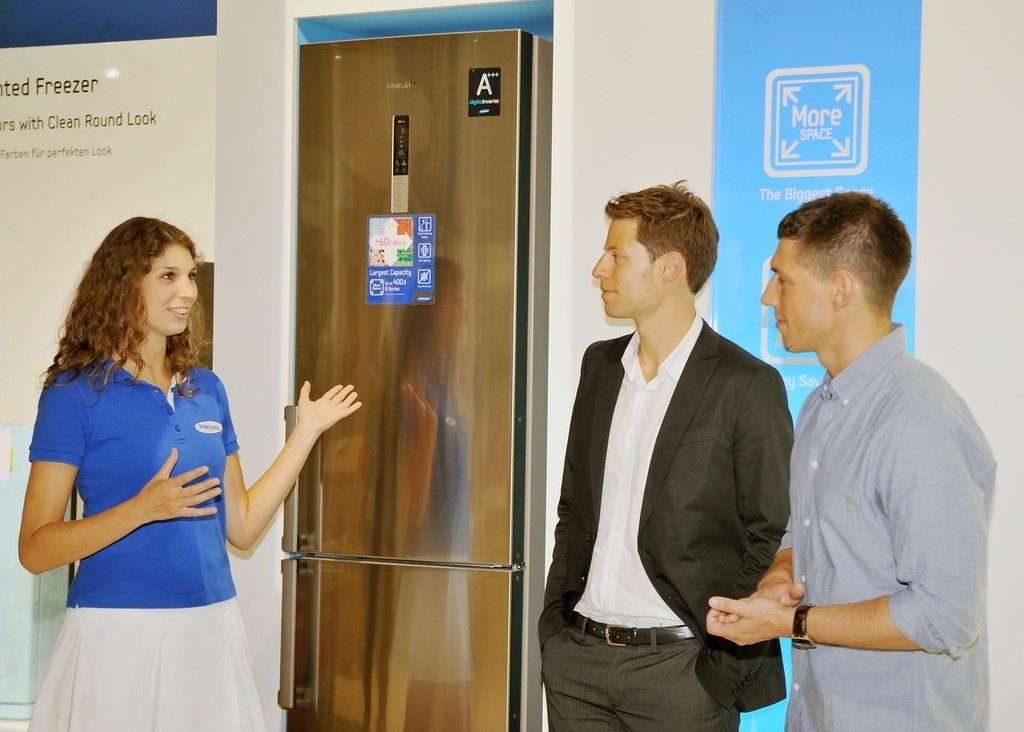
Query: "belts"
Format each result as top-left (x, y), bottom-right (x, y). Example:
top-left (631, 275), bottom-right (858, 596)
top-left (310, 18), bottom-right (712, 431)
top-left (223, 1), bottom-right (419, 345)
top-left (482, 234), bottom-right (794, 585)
top-left (567, 610), bottom-right (696, 647)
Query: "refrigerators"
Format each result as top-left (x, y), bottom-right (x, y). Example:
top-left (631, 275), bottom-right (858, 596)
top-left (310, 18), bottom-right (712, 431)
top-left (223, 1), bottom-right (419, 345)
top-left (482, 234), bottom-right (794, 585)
top-left (277, 29), bottom-right (544, 732)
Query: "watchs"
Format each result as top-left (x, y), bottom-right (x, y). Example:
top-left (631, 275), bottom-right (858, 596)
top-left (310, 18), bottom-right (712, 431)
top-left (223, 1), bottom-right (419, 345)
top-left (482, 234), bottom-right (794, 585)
top-left (791, 604), bottom-right (817, 649)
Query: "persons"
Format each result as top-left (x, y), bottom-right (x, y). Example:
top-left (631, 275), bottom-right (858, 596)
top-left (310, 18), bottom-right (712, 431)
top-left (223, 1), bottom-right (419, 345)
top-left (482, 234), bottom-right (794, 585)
top-left (707, 193), bottom-right (997, 732)
top-left (537, 185), bottom-right (795, 732)
top-left (19, 217), bottom-right (361, 732)
